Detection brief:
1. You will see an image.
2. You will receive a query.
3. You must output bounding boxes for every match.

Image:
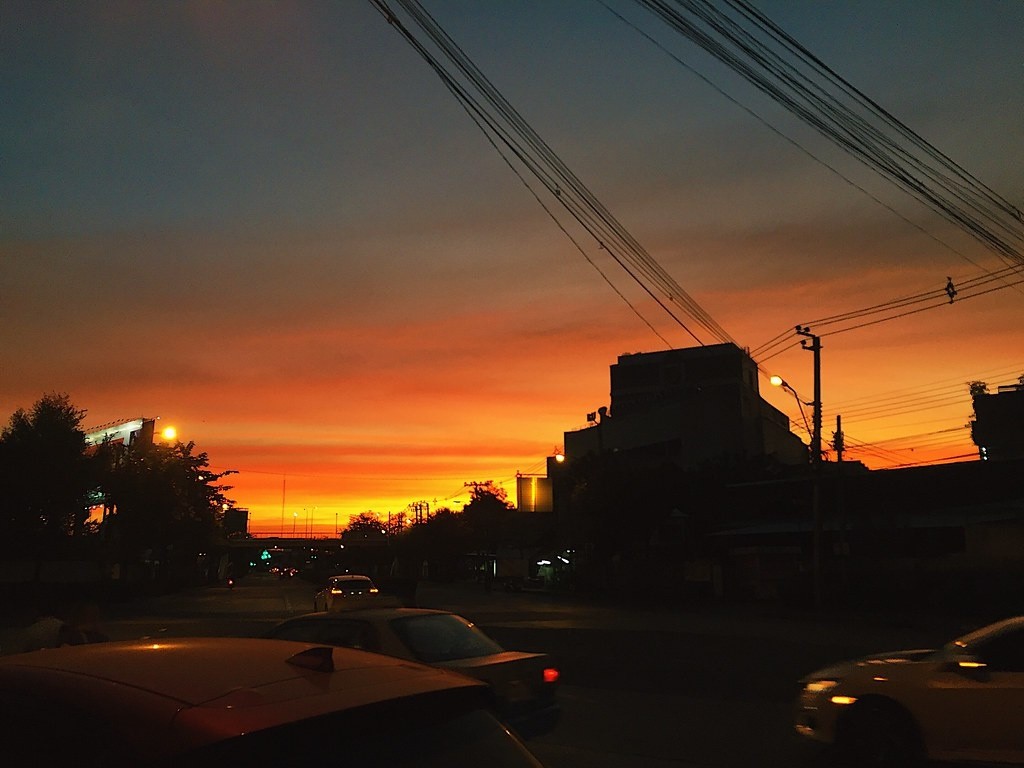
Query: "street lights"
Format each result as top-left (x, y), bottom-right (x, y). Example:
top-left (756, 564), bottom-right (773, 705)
top-left (770, 374), bottom-right (821, 611)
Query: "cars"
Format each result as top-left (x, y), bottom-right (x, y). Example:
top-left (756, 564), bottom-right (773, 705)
top-left (0, 639), bottom-right (539, 768)
top-left (792, 616), bottom-right (1024, 768)
top-left (313, 576), bottom-right (379, 613)
top-left (260, 576), bottom-right (558, 737)
